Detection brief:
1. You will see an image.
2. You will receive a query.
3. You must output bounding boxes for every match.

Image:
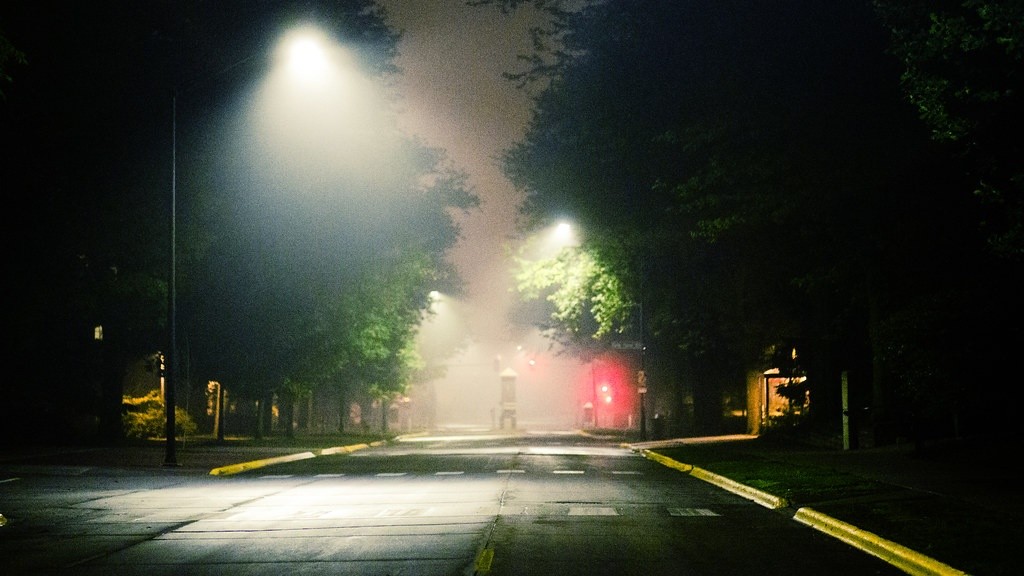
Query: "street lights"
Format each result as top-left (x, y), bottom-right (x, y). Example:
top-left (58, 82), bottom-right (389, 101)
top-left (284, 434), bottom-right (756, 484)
top-left (555, 217), bottom-right (648, 443)
top-left (164, 38), bottom-right (326, 471)
